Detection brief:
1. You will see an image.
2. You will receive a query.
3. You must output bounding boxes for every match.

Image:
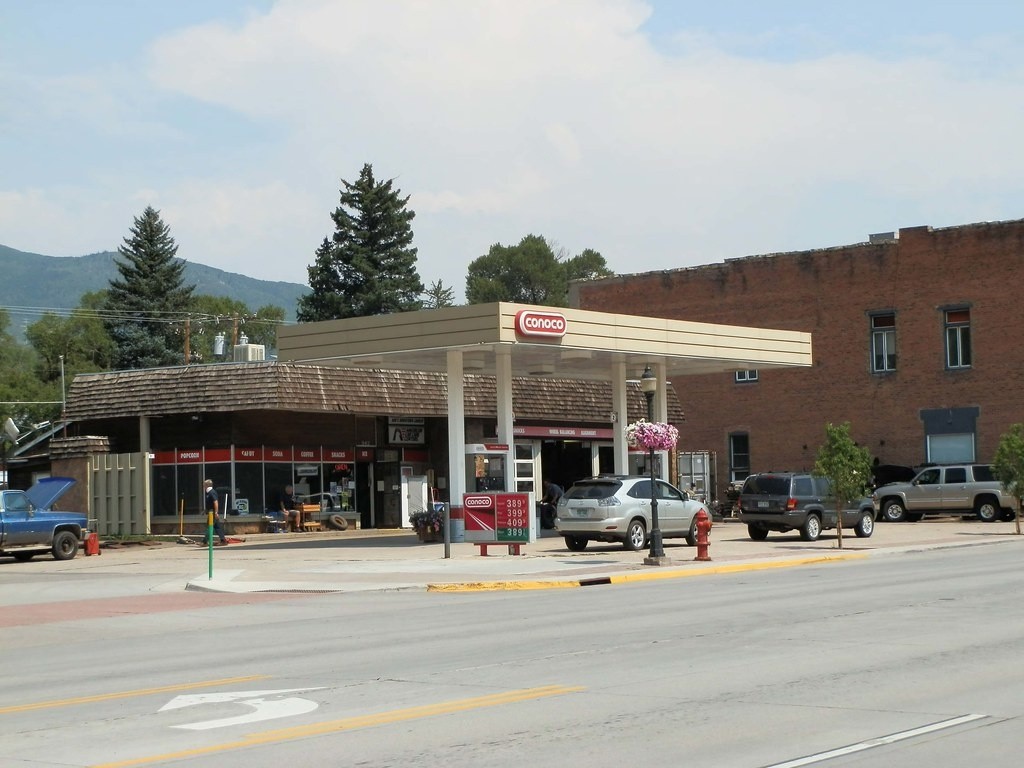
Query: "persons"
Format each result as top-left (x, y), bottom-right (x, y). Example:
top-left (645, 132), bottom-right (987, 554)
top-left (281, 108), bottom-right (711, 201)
top-left (538, 481), bottom-right (564, 508)
top-left (280, 485), bottom-right (304, 532)
top-left (198, 479), bottom-right (229, 547)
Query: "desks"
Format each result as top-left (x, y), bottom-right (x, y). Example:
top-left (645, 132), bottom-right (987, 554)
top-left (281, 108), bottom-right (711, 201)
top-left (309, 511), bottom-right (362, 532)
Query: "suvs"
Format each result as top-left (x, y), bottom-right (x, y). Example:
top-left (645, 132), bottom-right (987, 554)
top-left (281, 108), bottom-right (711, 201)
top-left (0, 474), bottom-right (89, 561)
top-left (872, 462), bottom-right (1022, 523)
top-left (554, 473), bottom-right (713, 552)
top-left (737, 471), bottom-right (878, 542)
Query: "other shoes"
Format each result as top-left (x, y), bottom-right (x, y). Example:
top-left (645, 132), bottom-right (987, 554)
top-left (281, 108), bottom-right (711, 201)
top-left (295, 527), bottom-right (302, 533)
top-left (195, 541), bottom-right (208, 547)
top-left (283, 527), bottom-right (288, 533)
top-left (213, 541), bottom-right (229, 546)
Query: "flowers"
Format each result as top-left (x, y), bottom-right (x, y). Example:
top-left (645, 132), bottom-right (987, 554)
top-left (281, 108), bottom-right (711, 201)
top-left (408, 507), bottom-right (443, 535)
top-left (624, 418), bottom-right (680, 453)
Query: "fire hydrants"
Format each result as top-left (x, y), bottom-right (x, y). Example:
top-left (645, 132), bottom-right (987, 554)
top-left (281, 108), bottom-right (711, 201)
top-left (694, 507), bottom-right (713, 561)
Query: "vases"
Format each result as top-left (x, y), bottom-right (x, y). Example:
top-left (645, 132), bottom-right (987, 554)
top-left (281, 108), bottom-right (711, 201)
top-left (419, 524), bottom-right (443, 543)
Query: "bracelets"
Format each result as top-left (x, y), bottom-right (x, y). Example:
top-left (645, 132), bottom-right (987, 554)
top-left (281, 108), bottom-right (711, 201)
top-left (214, 512), bottom-right (218, 515)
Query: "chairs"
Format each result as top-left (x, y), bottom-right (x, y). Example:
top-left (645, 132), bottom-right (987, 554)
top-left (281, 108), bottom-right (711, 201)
top-left (302, 502), bottom-right (322, 531)
